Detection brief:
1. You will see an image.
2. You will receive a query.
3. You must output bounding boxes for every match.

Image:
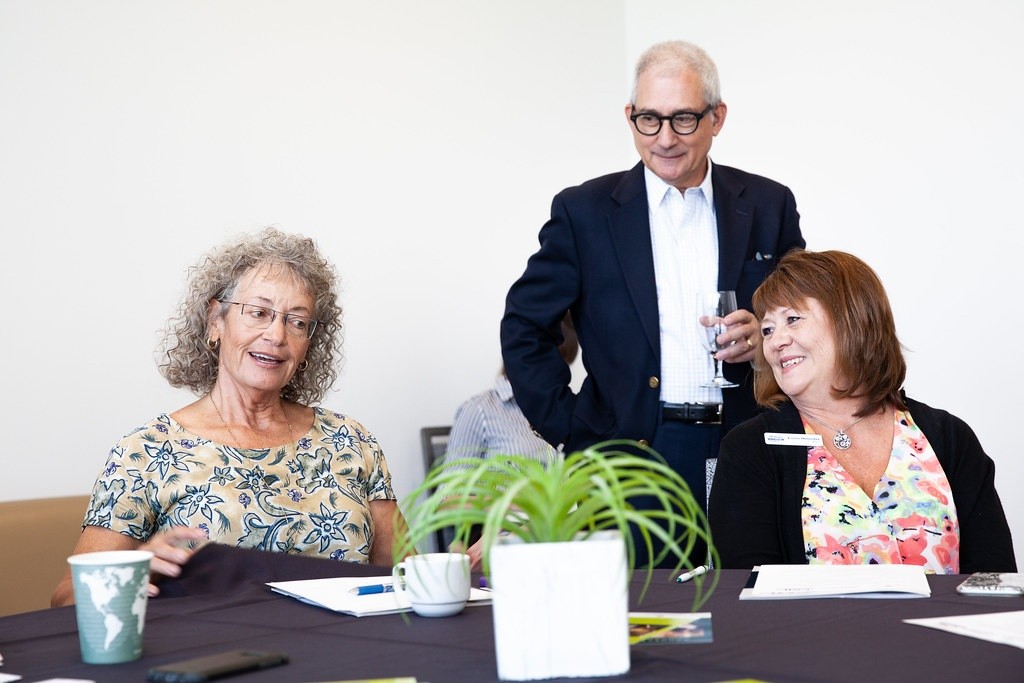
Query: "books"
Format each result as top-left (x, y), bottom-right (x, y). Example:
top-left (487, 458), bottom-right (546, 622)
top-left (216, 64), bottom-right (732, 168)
top-left (739, 564), bottom-right (931, 599)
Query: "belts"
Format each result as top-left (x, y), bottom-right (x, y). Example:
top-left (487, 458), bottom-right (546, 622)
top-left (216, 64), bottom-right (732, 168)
top-left (662, 403), bottom-right (723, 425)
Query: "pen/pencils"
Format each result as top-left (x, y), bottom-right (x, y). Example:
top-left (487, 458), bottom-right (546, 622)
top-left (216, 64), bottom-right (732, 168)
top-left (676, 565), bottom-right (714, 583)
top-left (347, 584), bottom-right (394, 596)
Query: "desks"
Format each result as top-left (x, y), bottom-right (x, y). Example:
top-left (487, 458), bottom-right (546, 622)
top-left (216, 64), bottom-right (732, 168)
top-left (0, 570), bottom-right (1024, 683)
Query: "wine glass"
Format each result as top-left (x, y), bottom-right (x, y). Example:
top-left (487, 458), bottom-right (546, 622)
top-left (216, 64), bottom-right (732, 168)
top-left (696, 292), bottom-right (742, 388)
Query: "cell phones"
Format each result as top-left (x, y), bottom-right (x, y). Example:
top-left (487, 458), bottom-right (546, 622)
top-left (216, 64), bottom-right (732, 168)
top-left (957, 571), bottom-right (1024, 597)
top-left (147, 649), bottom-right (290, 683)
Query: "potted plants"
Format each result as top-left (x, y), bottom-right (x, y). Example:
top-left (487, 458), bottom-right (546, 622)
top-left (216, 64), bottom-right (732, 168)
top-left (388, 436), bottom-right (722, 683)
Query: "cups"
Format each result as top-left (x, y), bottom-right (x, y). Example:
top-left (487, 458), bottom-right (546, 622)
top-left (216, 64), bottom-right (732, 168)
top-left (392, 553), bottom-right (470, 616)
top-left (68, 551), bottom-right (154, 663)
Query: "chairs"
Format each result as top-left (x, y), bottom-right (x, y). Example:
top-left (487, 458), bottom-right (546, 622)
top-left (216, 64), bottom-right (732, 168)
top-left (418, 426), bottom-right (453, 551)
top-left (0, 496), bottom-right (92, 619)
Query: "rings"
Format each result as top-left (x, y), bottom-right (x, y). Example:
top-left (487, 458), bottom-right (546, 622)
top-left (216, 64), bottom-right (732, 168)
top-left (746, 339), bottom-right (752, 351)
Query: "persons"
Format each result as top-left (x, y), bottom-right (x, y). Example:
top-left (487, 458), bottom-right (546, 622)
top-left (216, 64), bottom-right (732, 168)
top-left (51, 233), bottom-right (420, 607)
top-left (709, 250), bottom-right (1018, 575)
top-left (432, 324), bottom-right (579, 566)
top-left (500, 43), bottom-right (808, 569)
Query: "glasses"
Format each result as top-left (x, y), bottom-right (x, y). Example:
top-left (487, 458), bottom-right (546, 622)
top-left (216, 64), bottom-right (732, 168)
top-left (630, 103), bottom-right (713, 135)
top-left (219, 299), bottom-right (326, 342)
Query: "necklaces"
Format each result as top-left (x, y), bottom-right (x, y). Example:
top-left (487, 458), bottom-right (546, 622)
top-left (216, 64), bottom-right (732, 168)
top-left (801, 410), bottom-right (863, 449)
top-left (210, 390), bottom-right (300, 555)
